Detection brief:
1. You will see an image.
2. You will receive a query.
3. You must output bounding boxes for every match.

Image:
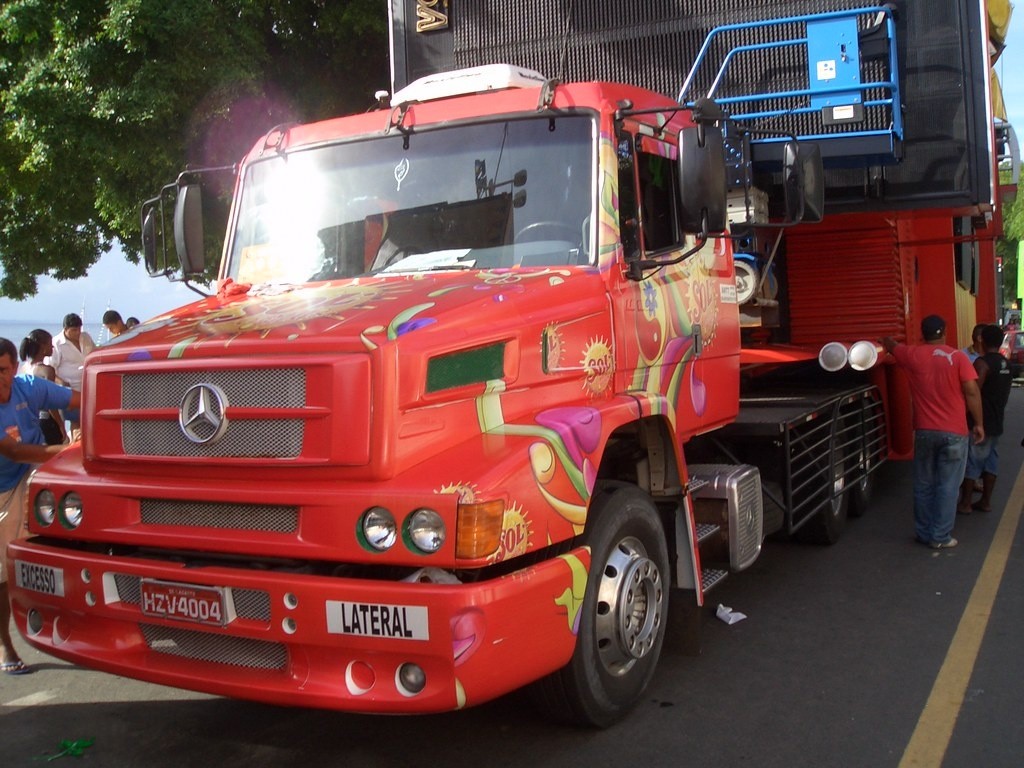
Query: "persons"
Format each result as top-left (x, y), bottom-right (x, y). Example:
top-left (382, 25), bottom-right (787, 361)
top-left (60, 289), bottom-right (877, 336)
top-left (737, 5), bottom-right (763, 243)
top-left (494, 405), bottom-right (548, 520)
top-left (876, 314), bottom-right (985, 549)
top-left (956, 323), bottom-right (1013, 514)
top-left (958, 323), bottom-right (991, 492)
top-left (43, 312), bottom-right (98, 443)
top-left (17, 328), bottom-right (70, 446)
top-left (0, 336), bottom-right (83, 677)
top-left (103, 310), bottom-right (139, 339)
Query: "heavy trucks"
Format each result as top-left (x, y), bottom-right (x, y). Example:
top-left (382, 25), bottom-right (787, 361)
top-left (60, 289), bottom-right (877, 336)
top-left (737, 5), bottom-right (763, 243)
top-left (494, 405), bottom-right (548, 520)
top-left (7, 0), bottom-right (1004, 725)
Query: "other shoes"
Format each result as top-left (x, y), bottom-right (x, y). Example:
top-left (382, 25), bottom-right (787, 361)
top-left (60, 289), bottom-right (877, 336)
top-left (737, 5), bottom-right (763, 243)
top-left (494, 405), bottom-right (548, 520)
top-left (912, 532), bottom-right (957, 549)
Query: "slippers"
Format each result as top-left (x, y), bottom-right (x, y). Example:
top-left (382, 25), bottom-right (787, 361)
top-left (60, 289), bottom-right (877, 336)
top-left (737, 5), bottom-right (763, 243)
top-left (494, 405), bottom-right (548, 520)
top-left (0, 657), bottom-right (31, 674)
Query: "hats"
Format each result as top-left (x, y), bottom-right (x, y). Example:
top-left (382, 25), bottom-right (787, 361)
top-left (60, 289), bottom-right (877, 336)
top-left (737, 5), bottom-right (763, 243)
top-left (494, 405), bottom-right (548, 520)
top-left (921, 315), bottom-right (945, 340)
